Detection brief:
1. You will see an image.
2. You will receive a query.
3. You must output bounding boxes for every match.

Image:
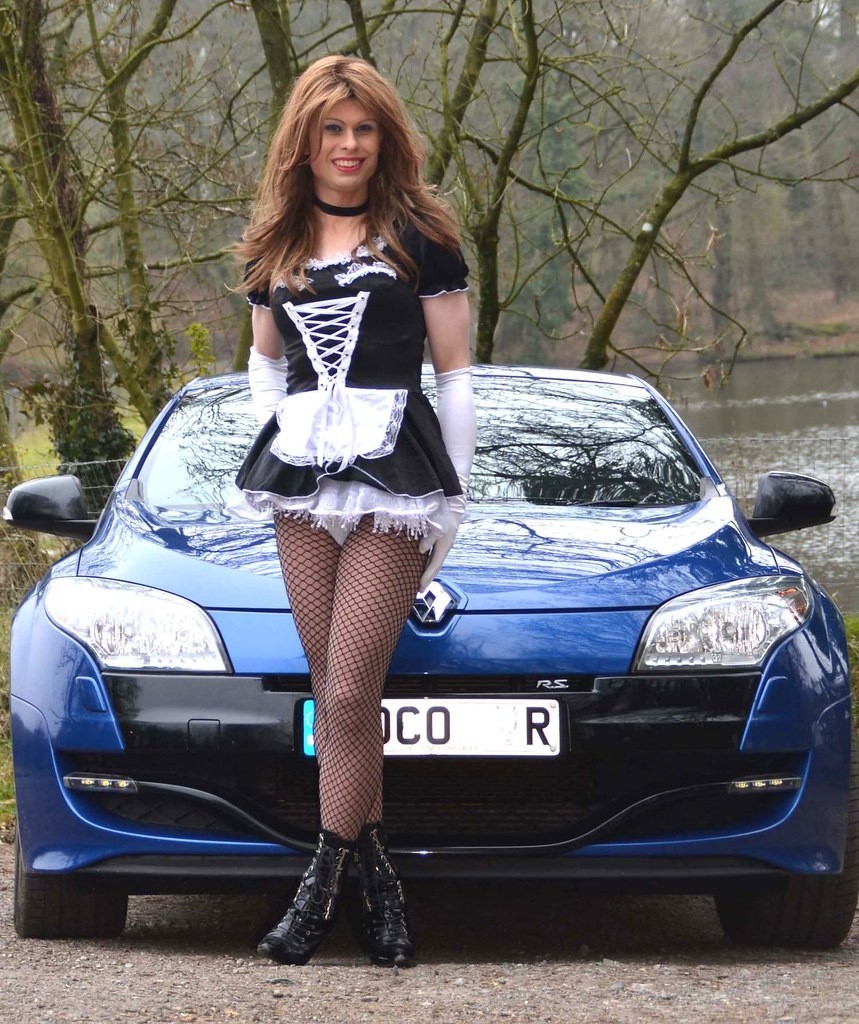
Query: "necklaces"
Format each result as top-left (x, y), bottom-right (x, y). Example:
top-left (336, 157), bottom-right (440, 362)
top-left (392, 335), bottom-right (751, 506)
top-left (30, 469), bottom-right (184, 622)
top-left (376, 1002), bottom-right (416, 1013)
top-left (310, 192), bottom-right (371, 216)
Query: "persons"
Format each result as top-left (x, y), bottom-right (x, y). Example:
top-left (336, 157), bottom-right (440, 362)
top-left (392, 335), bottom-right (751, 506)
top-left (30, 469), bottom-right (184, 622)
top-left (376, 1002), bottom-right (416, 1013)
top-left (217, 55), bottom-right (476, 969)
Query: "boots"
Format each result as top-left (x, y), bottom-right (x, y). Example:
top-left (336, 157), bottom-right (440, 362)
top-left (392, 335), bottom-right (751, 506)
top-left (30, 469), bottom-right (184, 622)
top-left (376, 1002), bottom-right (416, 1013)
top-left (352, 822), bottom-right (417, 968)
top-left (255, 828), bottom-right (352, 963)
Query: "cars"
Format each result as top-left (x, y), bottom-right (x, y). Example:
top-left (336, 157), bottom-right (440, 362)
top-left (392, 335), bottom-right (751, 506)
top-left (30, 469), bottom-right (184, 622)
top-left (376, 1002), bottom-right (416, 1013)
top-left (0, 365), bottom-right (859, 950)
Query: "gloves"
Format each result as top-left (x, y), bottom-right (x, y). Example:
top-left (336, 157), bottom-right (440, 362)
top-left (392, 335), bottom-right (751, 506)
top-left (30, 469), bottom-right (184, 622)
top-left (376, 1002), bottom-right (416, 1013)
top-left (417, 367), bottom-right (475, 592)
top-left (247, 345), bottom-right (289, 427)
